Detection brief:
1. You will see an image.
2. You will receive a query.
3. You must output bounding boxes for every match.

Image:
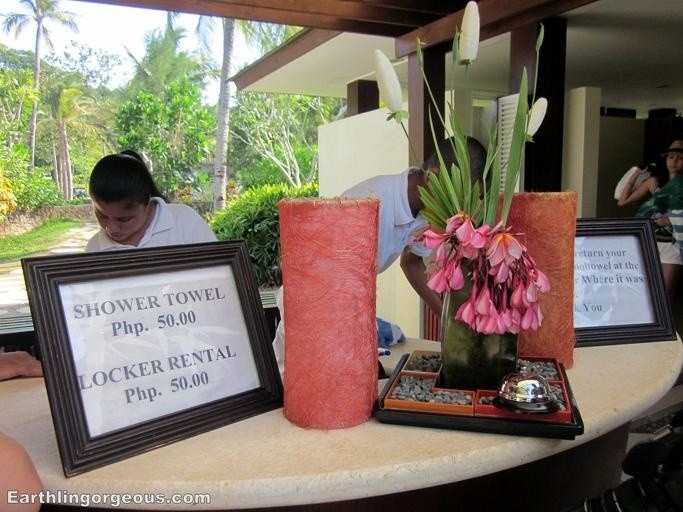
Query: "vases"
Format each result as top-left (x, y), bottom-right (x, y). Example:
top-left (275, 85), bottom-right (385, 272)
top-left (438, 268), bottom-right (519, 390)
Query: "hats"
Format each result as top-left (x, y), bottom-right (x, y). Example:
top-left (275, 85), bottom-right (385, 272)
top-left (661, 140), bottom-right (682, 156)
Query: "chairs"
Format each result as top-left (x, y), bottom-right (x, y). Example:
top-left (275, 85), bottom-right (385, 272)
top-left (555, 422), bottom-right (628, 512)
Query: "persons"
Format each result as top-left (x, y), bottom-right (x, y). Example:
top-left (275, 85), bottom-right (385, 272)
top-left (0, 349), bottom-right (43, 382)
top-left (336, 135), bottom-right (496, 321)
top-left (84, 148), bottom-right (221, 252)
top-left (615, 139), bottom-right (682, 294)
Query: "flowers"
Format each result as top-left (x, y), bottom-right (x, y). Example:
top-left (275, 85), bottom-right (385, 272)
top-left (372, 1), bottom-right (553, 337)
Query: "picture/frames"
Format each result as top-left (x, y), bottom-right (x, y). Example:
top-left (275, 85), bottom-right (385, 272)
top-left (570, 216), bottom-right (676, 346)
top-left (20, 237), bottom-right (286, 479)
